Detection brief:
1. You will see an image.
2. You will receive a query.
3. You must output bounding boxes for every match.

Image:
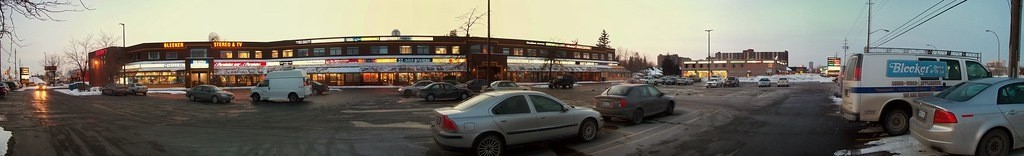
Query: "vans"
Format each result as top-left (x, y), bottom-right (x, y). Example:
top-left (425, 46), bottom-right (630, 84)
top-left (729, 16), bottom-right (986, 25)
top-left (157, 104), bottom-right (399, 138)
top-left (834, 51), bottom-right (996, 137)
top-left (249, 67), bottom-right (312, 103)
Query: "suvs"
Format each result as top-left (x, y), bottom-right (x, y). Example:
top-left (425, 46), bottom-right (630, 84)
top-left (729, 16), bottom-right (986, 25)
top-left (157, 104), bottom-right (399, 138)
top-left (549, 73), bottom-right (574, 89)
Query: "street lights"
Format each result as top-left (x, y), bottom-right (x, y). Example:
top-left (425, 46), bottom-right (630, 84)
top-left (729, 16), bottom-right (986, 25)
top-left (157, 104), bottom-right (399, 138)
top-left (705, 29), bottom-right (713, 81)
top-left (866, 28), bottom-right (889, 53)
top-left (119, 22), bottom-right (126, 85)
top-left (985, 29), bottom-right (1001, 78)
top-left (927, 44), bottom-right (939, 55)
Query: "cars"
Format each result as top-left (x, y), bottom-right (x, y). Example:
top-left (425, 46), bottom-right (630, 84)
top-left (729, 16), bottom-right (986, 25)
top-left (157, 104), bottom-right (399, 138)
top-left (594, 82), bottom-right (677, 125)
top-left (311, 81), bottom-right (329, 95)
top-left (777, 77), bottom-right (790, 87)
top-left (102, 83), bottom-right (130, 96)
top-left (757, 77), bottom-right (772, 87)
top-left (909, 75), bottom-right (1024, 156)
top-left (129, 82), bottom-right (149, 96)
top-left (397, 77), bottom-right (532, 103)
top-left (68, 82), bottom-right (92, 92)
top-left (0, 79), bottom-right (23, 95)
top-left (430, 89), bottom-right (605, 156)
top-left (625, 72), bottom-right (740, 89)
top-left (185, 84), bottom-right (235, 104)
